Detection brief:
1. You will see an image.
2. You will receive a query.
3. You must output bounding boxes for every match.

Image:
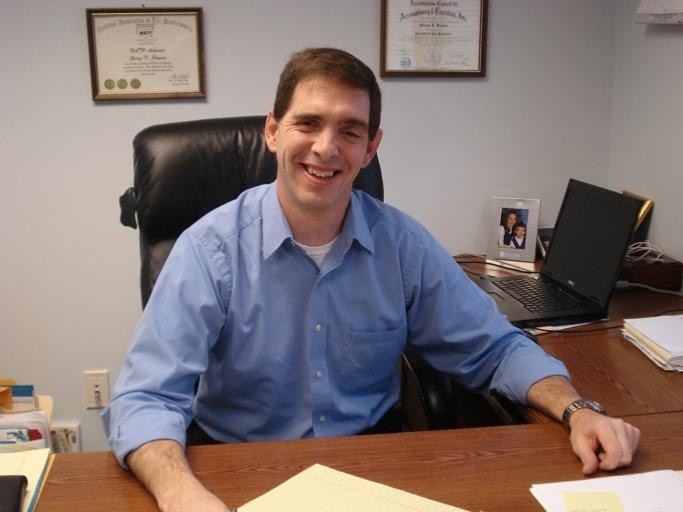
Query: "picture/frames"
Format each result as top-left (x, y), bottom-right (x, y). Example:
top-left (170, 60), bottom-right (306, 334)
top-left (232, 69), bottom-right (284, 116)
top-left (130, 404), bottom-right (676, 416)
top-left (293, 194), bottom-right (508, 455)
top-left (487, 197), bottom-right (541, 263)
top-left (85, 7), bottom-right (207, 102)
top-left (378, 0), bottom-right (489, 79)
top-left (622, 190), bottom-right (654, 234)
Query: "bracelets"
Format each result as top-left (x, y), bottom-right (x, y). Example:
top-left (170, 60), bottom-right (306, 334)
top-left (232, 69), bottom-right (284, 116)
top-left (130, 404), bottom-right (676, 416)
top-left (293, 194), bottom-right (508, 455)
top-left (562, 398), bottom-right (609, 428)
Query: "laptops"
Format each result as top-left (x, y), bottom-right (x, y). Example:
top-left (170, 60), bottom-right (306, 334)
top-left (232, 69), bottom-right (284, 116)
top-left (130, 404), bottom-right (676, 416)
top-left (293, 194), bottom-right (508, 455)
top-left (474, 177), bottom-right (641, 328)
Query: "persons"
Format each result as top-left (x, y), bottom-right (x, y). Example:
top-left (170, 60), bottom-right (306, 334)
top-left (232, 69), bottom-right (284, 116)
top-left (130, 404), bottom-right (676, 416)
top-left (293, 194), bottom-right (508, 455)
top-left (499, 212), bottom-right (517, 248)
top-left (99, 44), bottom-right (644, 511)
top-left (510, 223), bottom-right (525, 248)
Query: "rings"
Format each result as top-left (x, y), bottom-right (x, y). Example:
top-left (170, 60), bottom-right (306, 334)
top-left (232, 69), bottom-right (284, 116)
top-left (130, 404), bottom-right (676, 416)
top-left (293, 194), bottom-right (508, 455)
top-left (630, 449), bottom-right (636, 454)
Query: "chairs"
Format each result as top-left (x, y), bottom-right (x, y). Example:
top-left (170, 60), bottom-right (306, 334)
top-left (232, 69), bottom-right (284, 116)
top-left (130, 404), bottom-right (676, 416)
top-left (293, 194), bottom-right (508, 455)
top-left (119, 115), bottom-right (461, 430)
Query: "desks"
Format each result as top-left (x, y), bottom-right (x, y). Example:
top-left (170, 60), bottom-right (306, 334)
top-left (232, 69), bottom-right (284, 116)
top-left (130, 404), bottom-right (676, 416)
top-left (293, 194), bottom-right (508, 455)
top-left (396, 252), bottom-right (683, 422)
top-left (28, 409), bottom-right (683, 512)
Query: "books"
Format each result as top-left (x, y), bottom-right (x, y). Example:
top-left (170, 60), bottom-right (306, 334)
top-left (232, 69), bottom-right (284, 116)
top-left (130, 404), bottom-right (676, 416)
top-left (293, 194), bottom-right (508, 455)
top-left (618, 314), bottom-right (683, 373)
top-left (528, 468), bottom-right (683, 512)
top-left (0, 374), bottom-right (81, 512)
